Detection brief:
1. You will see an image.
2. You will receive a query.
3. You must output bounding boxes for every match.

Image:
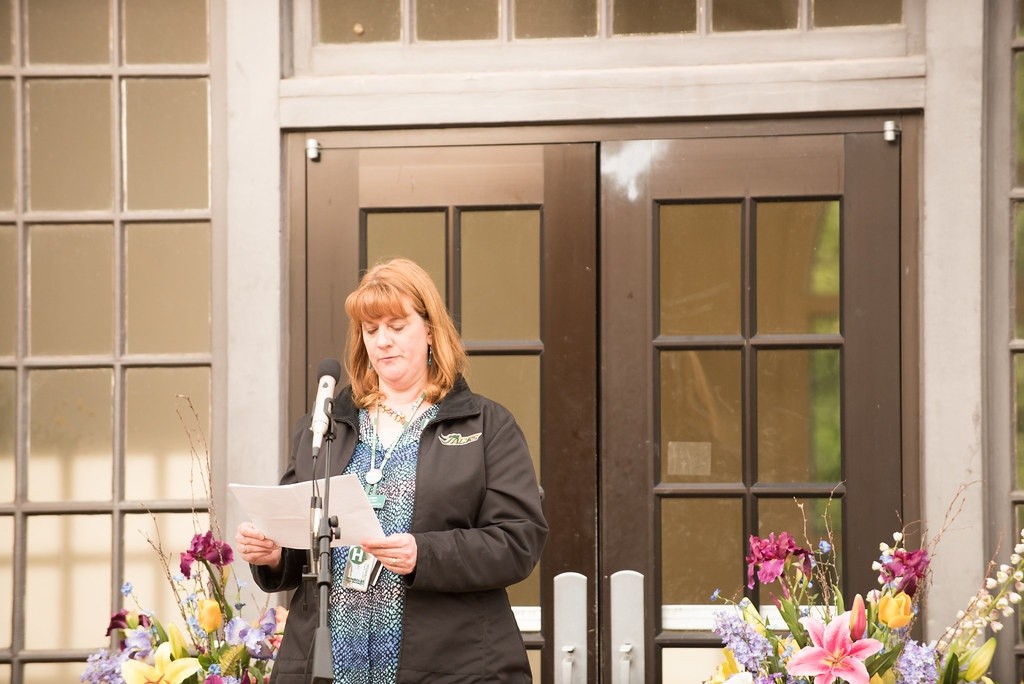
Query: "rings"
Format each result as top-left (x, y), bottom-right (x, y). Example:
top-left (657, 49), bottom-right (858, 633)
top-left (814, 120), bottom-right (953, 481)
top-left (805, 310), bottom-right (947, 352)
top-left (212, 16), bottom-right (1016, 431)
top-left (242, 544), bottom-right (248, 554)
top-left (391, 557), bottom-right (398, 566)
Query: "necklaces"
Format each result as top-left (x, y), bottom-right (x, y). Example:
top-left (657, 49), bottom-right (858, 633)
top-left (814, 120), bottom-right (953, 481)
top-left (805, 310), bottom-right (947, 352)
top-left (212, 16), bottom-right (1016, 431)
top-left (367, 384), bottom-right (432, 431)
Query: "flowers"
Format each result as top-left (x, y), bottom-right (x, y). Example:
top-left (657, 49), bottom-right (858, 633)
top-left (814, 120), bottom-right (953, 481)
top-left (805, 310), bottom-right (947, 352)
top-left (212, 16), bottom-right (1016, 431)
top-left (701, 476), bottom-right (1024, 684)
top-left (79, 394), bottom-right (294, 684)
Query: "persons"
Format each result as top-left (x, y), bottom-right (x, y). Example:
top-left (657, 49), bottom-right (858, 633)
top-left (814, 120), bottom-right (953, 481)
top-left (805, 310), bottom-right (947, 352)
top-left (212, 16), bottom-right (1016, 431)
top-left (233, 256), bottom-right (551, 684)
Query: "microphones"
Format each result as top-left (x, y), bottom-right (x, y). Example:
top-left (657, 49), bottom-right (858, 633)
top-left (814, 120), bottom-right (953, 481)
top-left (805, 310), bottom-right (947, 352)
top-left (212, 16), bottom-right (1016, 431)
top-left (310, 358), bottom-right (342, 458)
